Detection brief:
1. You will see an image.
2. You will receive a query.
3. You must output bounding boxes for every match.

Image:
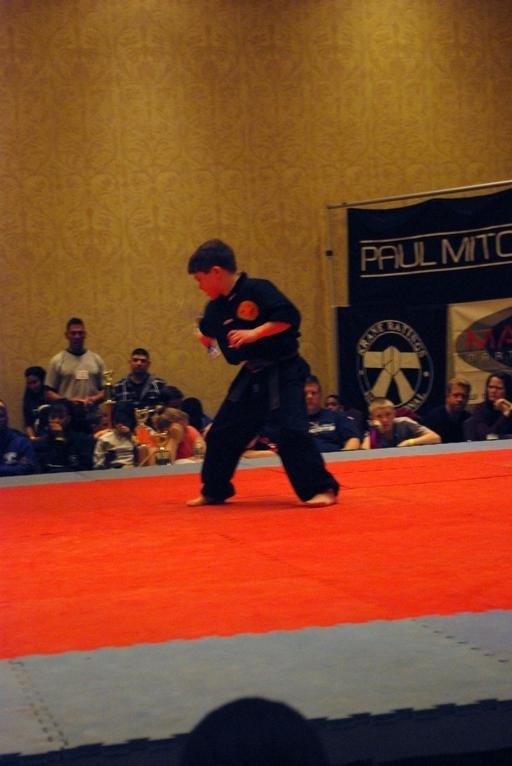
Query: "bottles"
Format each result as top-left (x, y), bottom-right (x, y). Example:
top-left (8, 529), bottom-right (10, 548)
top-left (193, 438), bottom-right (202, 461)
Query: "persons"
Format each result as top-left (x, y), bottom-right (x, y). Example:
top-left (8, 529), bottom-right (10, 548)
top-left (243, 370), bottom-right (512, 458)
top-left (177, 696), bottom-right (330, 766)
top-left (0, 316), bottom-right (213, 476)
top-left (186, 237), bottom-right (341, 507)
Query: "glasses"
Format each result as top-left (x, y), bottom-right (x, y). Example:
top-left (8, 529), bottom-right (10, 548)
top-left (148, 407), bottom-right (166, 416)
top-left (131, 358), bottom-right (146, 364)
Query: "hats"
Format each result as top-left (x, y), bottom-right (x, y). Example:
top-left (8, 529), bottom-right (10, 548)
top-left (143, 392), bottom-right (160, 407)
top-left (141, 392), bottom-right (161, 404)
